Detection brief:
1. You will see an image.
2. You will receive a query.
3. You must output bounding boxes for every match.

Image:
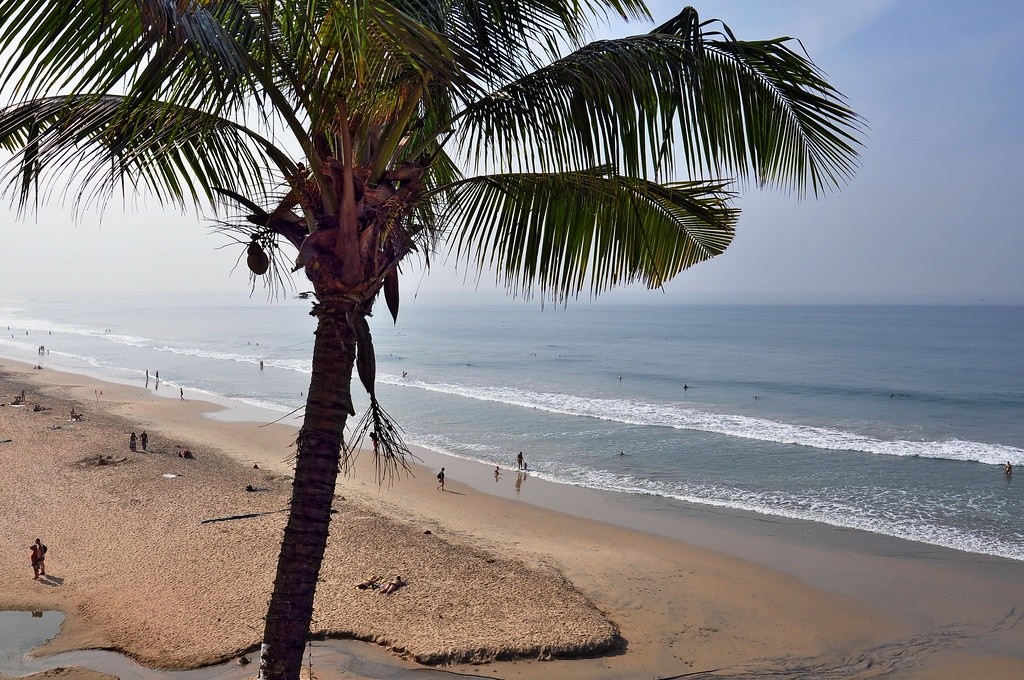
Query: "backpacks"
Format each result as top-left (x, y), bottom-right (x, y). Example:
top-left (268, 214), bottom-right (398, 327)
top-left (437, 472), bottom-right (441, 479)
top-left (41, 544), bottom-right (48, 554)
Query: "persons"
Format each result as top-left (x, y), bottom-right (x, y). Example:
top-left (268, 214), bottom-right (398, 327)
top-left (130, 432), bottom-right (138, 451)
top-left (70, 408), bottom-right (82, 420)
top-left (353, 574), bottom-right (383, 591)
top-left (180, 387), bottom-right (184, 400)
top-left (30, 545), bottom-right (40, 580)
top-left (11, 387), bottom-right (42, 412)
top-left (145, 369), bottom-right (148, 380)
top-left (141, 430), bottom-right (148, 450)
top-left (494, 466), bottom-right (499, 475)
top-left (35, 538), bottom-right (45, 573)
top-left (156, 370), bottom-right (159, 381)
top-left (378, 575), bottom-right (403, 595)
top-left (1004, 461), bottom-right (1012, 476)
top-left (516, 451), bottom-right (523, 470)
top-left (437, 467), bottom-right (446, 491)
